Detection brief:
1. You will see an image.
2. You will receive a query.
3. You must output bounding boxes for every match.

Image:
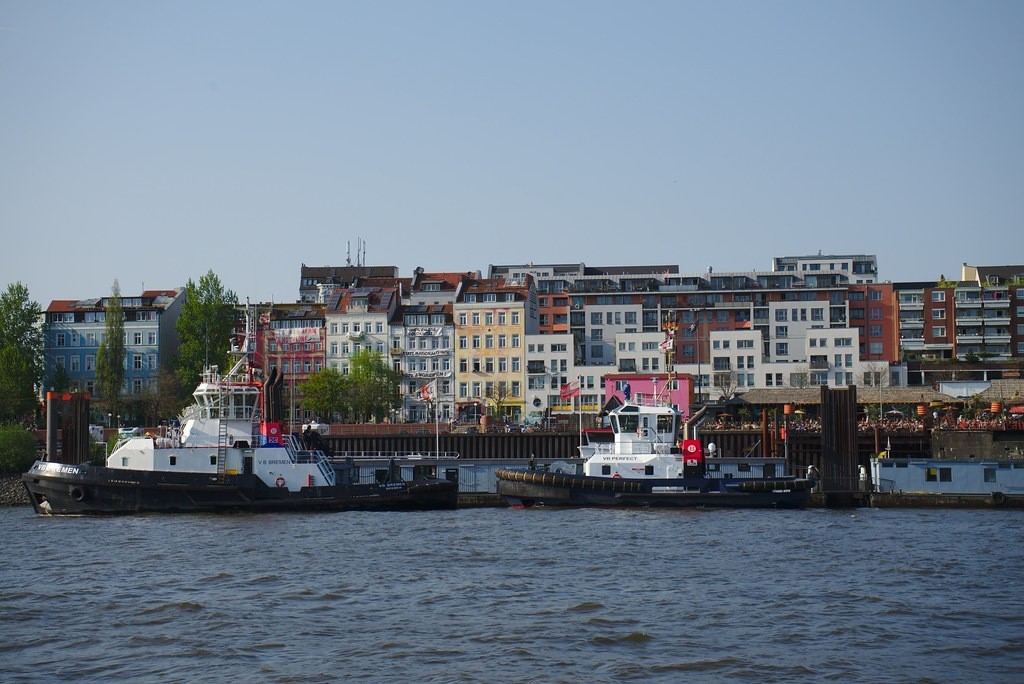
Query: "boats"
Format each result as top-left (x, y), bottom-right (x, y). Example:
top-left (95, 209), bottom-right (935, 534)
top-left (19, 302), bottom-right (460, 517)
top-left (495, 321), bottom-right (820, 510)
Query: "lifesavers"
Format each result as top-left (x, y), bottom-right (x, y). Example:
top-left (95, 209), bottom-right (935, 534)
top-left (991, 492), bottom-right (1005, 506)
top-left (554, 468), bottom-right (563, 474)
top-left (70, 486), bottom-right (85, 503)
top-left (613, 473), bottom-right (622, 479)
top-left (274, 476), bottom-right (286, 487)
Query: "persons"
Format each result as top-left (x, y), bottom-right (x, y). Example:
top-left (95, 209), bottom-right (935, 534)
top-left (705, 410), bottom-right (1024, 434)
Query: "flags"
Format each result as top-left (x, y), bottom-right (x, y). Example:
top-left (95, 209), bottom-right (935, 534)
top-left (687, 319), bottom-right (697, 334)
top-left (560, 380), bottom-right (580, 399)
top-left (659, 339), bottom-right (673, 350)
top-left (415, 379), bottom-right (436, 401)
top-left (662, 313), bottom-right (680, 338)
